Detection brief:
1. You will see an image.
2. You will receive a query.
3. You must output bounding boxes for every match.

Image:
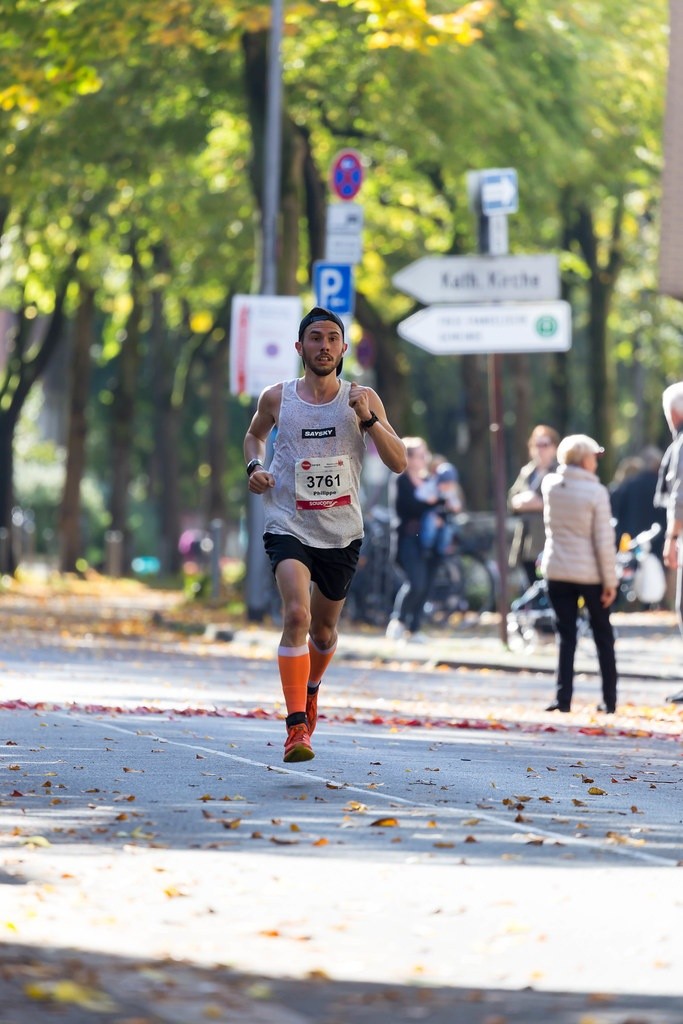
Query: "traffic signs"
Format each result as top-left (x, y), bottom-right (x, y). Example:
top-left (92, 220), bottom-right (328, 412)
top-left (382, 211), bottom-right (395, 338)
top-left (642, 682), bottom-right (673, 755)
top-left (392, 253), bottom-right (575, 361)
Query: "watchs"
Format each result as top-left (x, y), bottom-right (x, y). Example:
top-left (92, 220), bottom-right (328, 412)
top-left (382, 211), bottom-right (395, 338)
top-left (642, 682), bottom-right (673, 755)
top-left (359, 410), bottom-right (380, 431)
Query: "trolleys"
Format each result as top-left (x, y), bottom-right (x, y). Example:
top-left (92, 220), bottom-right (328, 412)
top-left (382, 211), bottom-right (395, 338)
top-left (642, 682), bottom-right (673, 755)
top-left (509, 525), bottom-right (660, 644)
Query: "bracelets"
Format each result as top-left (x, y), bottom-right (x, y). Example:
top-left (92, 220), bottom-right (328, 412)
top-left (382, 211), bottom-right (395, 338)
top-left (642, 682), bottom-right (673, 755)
top-left (246, 459), bottom-right (264, 476)
top-left (665, 534), bottom-right (678, 539)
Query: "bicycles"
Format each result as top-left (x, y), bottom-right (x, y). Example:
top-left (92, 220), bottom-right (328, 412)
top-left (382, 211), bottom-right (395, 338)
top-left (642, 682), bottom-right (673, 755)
top-left (426, 512), bottom-right (499, 632)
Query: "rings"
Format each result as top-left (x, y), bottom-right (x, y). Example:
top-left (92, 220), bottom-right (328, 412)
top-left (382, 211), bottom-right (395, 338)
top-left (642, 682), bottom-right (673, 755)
top-left (253, 485), bottom-right (255, 490)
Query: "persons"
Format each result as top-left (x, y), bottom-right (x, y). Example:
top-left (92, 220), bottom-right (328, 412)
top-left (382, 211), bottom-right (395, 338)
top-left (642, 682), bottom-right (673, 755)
top-left (652, 382), bottom-right (683, 702)
top-left (506, 425), bottom-right (560, 637)
top-left (245, 307), bottom-right (409, 761)
top-left (539, 434), bottom-right (621, 715)
top-left (357, 433), bottom-right (466, 645)
top-left (606, 444), bottom-right (668, 572)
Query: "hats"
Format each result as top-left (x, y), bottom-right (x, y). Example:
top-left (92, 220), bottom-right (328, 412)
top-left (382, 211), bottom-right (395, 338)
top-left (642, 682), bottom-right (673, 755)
top-left (557, 434), bottom-right (604, 464)
top-left (299, 307), bottom-right (344, 376)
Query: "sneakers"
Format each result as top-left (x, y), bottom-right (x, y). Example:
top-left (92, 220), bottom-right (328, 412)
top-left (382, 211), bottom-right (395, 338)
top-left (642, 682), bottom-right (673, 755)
top-left (306, 680), bottom-right (321, 737)
top-left (283, 712), bottom-right (315, 762)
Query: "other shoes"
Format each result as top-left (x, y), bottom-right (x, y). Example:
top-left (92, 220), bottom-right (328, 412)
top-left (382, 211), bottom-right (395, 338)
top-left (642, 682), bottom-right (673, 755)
top-left (666, 690), bottom-right (683, 702)
top-left (597, 705), bottom-right (615, 713)
top-left (386, 621), bottom-right (428, 643)
top-left (545, 705), bottom-right (570, 712)
